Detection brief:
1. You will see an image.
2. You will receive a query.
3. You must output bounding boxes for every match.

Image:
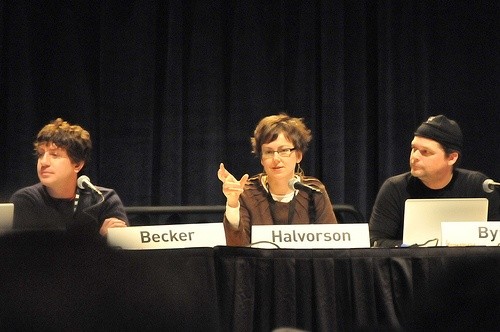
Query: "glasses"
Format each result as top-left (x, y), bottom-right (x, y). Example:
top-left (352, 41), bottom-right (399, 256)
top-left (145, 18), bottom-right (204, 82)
top-left (260, 147), bottom-right (296, 158)
top-left (36, 152), bottom-right (70, 158)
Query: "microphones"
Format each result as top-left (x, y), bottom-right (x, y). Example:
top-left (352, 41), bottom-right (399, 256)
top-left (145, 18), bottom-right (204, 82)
top-left (77, 175), bottom-right (103, 196)
top-left (288, 178), bottom-right (322, 195)
top-left (483, 179), bottom-right (500, 193)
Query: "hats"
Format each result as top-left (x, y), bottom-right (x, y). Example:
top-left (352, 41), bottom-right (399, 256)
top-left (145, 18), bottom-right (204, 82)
top-left (414, 115), bottom-right (463, 151)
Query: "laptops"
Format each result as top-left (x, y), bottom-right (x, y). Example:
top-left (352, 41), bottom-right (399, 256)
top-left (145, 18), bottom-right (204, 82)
top-left (402, 198), bottom-right (488, 246)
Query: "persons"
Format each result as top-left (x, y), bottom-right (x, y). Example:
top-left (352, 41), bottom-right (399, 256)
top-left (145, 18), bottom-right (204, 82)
top-left (9, 118), bottom-right (129, 236)
top-left (369, 115), bottom-right (500, 248)
top-left (218, 114), bottom-right (337, 248)
